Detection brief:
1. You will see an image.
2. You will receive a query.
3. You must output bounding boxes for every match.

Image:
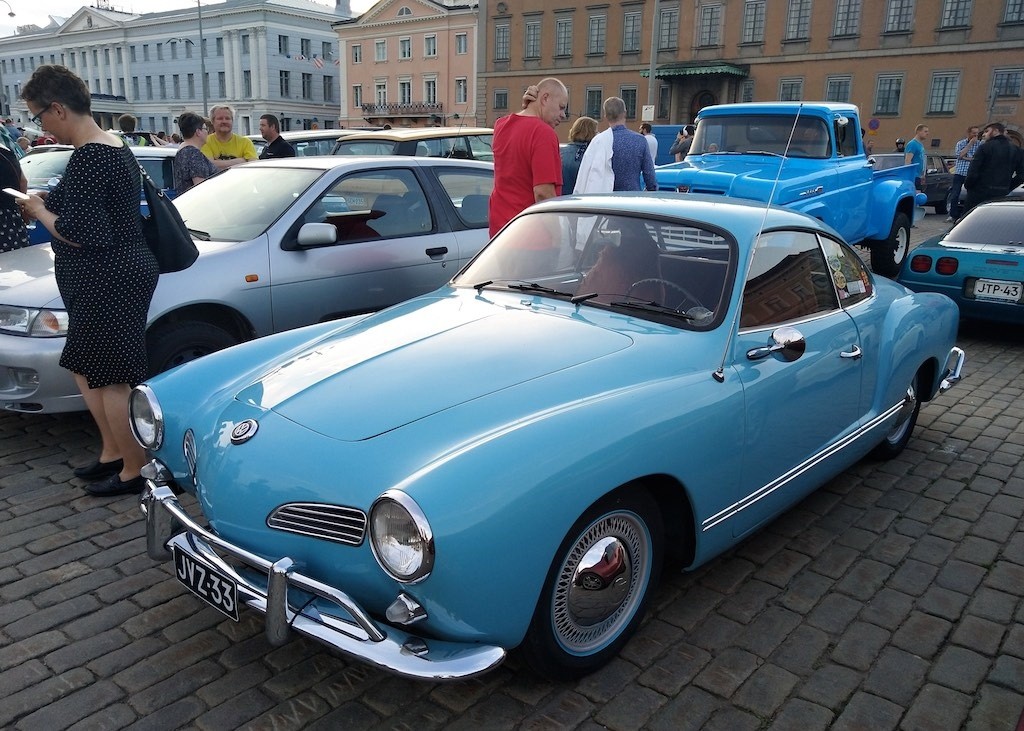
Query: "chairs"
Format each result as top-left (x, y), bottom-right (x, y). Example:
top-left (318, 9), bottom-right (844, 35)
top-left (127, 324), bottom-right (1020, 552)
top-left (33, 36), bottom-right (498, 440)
top-left (369, 191), bottom-right (427, 232)
top-left (577, 243), bottom-right (655, 307)
top-left (461, 195), bottom-right (491, 223)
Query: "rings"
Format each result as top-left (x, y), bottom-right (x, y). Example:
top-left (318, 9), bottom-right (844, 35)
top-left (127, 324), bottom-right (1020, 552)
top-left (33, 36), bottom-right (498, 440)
top-left (21, 204), bottom-right (24, 213)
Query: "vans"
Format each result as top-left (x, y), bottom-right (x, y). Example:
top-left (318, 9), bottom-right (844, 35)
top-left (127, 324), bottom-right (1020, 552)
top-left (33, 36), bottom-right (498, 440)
top-left (651, 124), bottom-right (695, 167)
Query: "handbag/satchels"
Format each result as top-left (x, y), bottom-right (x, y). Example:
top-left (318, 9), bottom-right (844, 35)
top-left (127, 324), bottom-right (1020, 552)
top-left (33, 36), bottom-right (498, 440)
top-left (133, 155), bottom-right (199, 274)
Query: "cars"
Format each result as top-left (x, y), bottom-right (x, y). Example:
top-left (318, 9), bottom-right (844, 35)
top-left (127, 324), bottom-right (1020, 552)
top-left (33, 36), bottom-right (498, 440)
top-left (243, 126), bottom-right (569, 213)
top-left (125, 191), bottom-right (967, 687)
top-left (19, 144), bottom-right (180, 246)
top-left (925, 153), bottom-right (958, 215)
top-left (0, 157), bottom-right (580, 415)
top-left (899, 197), bottom-right (1024, 322)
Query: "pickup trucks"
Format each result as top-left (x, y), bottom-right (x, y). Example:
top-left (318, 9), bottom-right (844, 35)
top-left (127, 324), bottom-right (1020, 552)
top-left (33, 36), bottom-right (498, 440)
top-left (598, 102), bottom-right (928, 279)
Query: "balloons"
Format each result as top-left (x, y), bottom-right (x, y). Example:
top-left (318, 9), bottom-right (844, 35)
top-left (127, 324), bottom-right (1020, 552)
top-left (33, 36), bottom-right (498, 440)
top-left (915, 193), bottom-right (928, 206)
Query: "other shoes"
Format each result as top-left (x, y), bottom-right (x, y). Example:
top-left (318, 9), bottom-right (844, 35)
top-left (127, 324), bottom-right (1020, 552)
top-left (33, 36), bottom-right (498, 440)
top-left (85, 473), bottom-right (145, 497)
top-left (943, 216), bottom-right (955, 222)
top-left (911, 225), bottom-right (918, 228)
top-left (74, 457), bottom-right (124, 480)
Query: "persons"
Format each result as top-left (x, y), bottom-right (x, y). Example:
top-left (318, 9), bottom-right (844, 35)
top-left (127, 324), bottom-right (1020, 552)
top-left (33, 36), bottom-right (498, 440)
top-left (173, 111), bottom-right (221, 195)
top-left (489, 76), bottom-right (569, 279)
top-left (945, 122), bottom-right (1024, 224)
top-left (892, 138), bottom-right (905, 152)
top-left (904, 124), bottom-right (929, 193)
top-left (200, 104), bottom-right (258, 170)
top-left (669, 125), bottom-right (718, 163)
top-left (558, 116), bottom-right (600, 196)
top-left (0, 118), bottom-right (30, 150)
top-left (638, 122), bottom-right (658, 166)
top-left (0, 144), bottom-right (27, 253)
top-left (118, 114), bottom-right (182, 148)
top-left (865, 139), bottom-right (875, 155)
top-left (572, 96), bottom-right (659, 251)
top-left (15, 64), bottom-right (160, 498)
top-left (259, 114), bottom-right (295, 159)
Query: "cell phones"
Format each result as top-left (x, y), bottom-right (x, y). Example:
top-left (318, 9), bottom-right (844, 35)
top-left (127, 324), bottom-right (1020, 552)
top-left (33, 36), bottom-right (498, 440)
top-left (2, 187), bottom-right (31, 199)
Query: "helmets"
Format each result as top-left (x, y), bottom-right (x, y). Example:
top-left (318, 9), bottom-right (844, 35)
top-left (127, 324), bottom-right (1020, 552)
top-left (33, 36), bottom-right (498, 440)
top-left (895, 138), bottom-right (905, 147)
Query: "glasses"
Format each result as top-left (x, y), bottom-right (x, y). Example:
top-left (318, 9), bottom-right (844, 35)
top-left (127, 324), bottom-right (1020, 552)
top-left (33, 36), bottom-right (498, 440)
top-left (31, 104), bottom-right (51, 127)
top-left (201, 127), bottom-right (210, 132)
